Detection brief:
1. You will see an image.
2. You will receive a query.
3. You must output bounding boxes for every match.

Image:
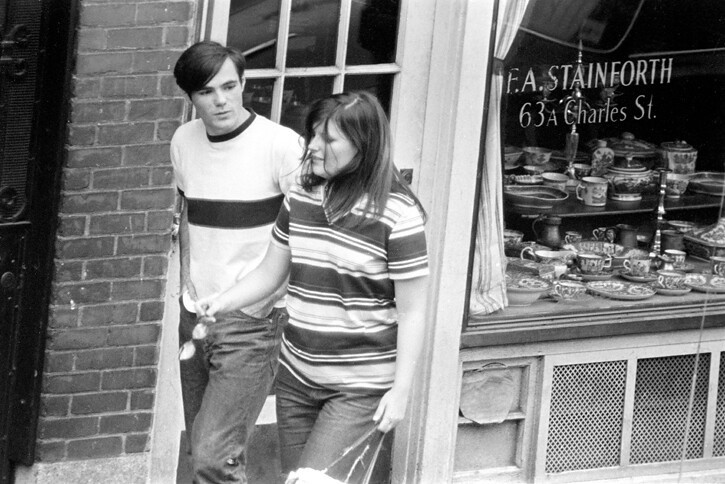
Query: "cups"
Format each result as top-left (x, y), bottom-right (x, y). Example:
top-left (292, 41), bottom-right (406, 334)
top-left (571, 163), bottom-right (591, 176)
top-left (655, 174), bottom-right (691, 198)
top-left (623, 258), bottom-right (652, 276)
top-left (577, 254), bottom-right (611, 274)
top-left (554, 283), bottom-right (586, 302)
top-left (542, 163), bottom-right (609, 206)
top-left (656, 250), bottom-right (687, 273)
top-left (709, 256), bottom-right (725, 276)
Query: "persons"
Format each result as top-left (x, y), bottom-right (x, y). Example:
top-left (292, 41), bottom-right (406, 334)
top-left (169, 40), bottom-right (311, 483)
top-left (195, 91), bottom-right (429, 484)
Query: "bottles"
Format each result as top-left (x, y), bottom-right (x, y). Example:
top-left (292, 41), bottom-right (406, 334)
top-left (589, 132), bottom-right (697, 201)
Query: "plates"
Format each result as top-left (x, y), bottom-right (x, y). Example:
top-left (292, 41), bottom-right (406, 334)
top-left (502, 162), bottom-right (558, 172)
top-left (690, 171), bottom-right (725, 196)
top-left (504, 220), bottom-right (725, 305)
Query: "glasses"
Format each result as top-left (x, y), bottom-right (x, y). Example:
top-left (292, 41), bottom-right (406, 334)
top-left (175, 322), bottom-right (209, 361)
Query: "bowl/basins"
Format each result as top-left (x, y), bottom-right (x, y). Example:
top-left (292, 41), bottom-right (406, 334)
top-left (504, 173), bottom-right (569, 209)
top-left (504, 276), bottom-right (553, 305)
top-left (522, 146), bottom-right (553, 165)
top-left (502, 146), bottom-right (523, 166)
top-left (552, 150), bottom-right (590, 171)
top-left (564, 240), bottom-right (624, 257)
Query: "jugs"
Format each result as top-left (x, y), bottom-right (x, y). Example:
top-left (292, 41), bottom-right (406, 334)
top-left (605, 224), bottom-right (641, 250)
top-left (532, 214), bottom-right (565, 248)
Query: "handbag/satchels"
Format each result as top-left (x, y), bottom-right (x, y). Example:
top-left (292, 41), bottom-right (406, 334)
top-left (284, 425), bottom-right (386, 484)
